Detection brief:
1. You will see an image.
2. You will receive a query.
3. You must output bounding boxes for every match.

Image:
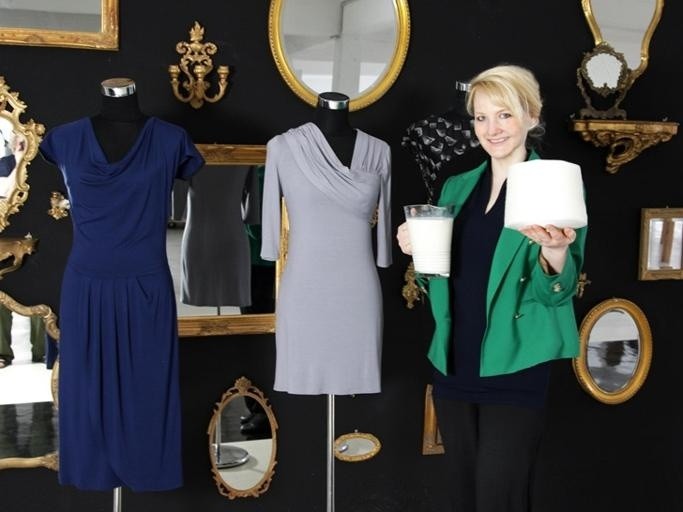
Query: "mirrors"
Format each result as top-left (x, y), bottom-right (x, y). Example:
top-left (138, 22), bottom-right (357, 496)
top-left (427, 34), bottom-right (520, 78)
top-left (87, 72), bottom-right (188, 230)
top-left (335, 433), bottom-right (382, 462)
top-left (0, 2), bottom-right (118, 51)
top-left (637, 206), bottom-right (683, 282)
top-left (0, 288), bottom-right (64, 481)
top-left (574, 0), bottom-right (664, 122)
top-left (572, 298), bottom-right (653, 405)
top-left (265, 0), bottom-right (411, 112)
top-left (167, 143), bottom-right (288, 337)
top-left (206, 376), bottom-right (279, 501)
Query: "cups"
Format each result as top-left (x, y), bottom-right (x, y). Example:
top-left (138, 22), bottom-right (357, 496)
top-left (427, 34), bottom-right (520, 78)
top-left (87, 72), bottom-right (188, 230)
top-left (403, 202), bottom-right (454, 277)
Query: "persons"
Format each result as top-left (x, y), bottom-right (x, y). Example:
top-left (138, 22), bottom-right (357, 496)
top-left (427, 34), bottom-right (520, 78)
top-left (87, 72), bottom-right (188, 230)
top-left (397, 63), bottom-right (591, 512)
top-left (257, 91), bottom-right (397, 401)
top-left (237, 395), bottom-right (272, 436)
top-left (37, 75), bottom-right (205, 496)
top-left (179, 164), bottom-right (263, 313)
top-left (0, 302), bottom-right (48, 371)
